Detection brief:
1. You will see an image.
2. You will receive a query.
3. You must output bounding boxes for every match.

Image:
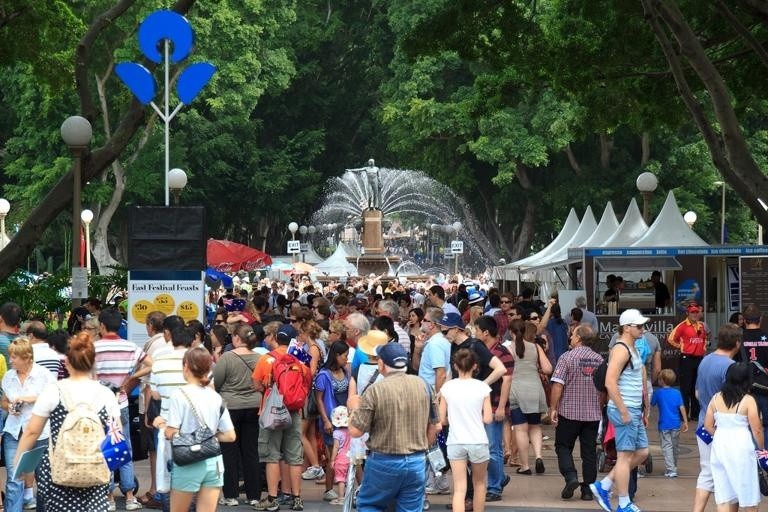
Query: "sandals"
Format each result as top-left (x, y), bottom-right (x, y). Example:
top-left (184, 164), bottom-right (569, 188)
top-left (136, 492), bottom-right (154, 505)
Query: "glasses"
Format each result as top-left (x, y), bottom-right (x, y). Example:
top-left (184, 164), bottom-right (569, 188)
top-left (8, 340), bottom-right (17, 343)
top-left (508, 313), bottom-right (517, 317)
top-left (500, 301), bottom-right (511, 304)
top-left (529, 317), bottom-right (537, 320)
top-left (313, 305), bottom-right (318, 309)
top-left (356, 308), bottom-right (365, 310)
top-left (423, 318), bottom-right (431, 322)
top-left (328, 330), bottom-right (336, 334)
top-left (442, 329), bottom-right (451, 335)
top-left (290, 320), bottom-right (298, 324)
top-left (477, 312), bottom-right (484, 315)
top-left (344, 327), bottom-right (354, 330)
top-left (328, 297), bottom-right (333, 299)
top-left (627, 324), bottom-right (643, 329)
top-left (215, 320), bottom-right (224, 324)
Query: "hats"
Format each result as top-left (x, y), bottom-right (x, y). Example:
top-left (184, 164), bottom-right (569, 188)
top-left (431, 313), bottom-right (465, 331)
top-left (287, 289), bottom-right (294, 293)
top-left (377, 342), bottom-right (408, 368)
top-left (358, 330), bottom-right (388, 356)
top-left (688, 304), bottom-right (699, 313)
top-left (228, 299), bottom-right (247, 310)
top-left (331, 407), bottom-right (352, 428)
top-left (619, 309), bottom-right (650, 326)
top-left (349, 297), bottom-right (368, 307)
top-left (468, 294), bottom-right (484, 304)
top-left (277, 325), bottom-right (299, 339)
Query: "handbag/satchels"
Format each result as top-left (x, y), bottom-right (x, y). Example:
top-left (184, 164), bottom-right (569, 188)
top-left (171, 387), bottom-right (222, 466)
top-left (258, 382), bottom-right (291, 429)
top-left (535, 344), bottom-right (549, 386)
top-left (740, 342), bottom-right (767, 393)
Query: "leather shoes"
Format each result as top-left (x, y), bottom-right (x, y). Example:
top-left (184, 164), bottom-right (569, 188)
top-left (465, 498), bottom-right (474, 511)
top-left (510, 460), bottom-right (522, 466)
top-left (517, 468), bottom-right (531, 474)
top-left (682, 416), bottom-right (690, 420)
top-left (503, 452), bottom-right (511, 464)
top-left (536, 458), bottom-right (545, 473)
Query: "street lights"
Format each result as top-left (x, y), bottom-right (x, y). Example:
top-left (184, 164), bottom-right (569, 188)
top-left (322, 224), bottom-right (327, 233)
top-left (61, 115), bottom-right (92, 307)
top-left (426, 223), bottom-right (431, 260)
top-left (636, 172), bottom-right (659, 224)
top-left (332, 223), bottom-right (337, 232)
top-left (328, 224), bottom-right (333, 234)
top-left (300, 226), bottom-right (307, 264)
top-left (431, 224), bottom-right (436, 264)
top-left (289, 222), bottom-right (298, 261)
top-left (441, 224), bottom-right (448, 248)
top-left (168, 168), bottom-right (187, 204)
top-left (0, 198), bottom-right (10, 249)
top-left (453, 222), bottom-right (462, 274)
top-left (446, 225), bottom-right (454, 272)
top-left (686, 213), bottom-right (694, 228)
top-left (309, 226), bottom-right (315, 241)
top-left (316, 225), bottom-right (322, 233)
top-left (81, 209), bottom-right (94, 274)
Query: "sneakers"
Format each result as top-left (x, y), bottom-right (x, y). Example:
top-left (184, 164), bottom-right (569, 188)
top-left (617, 503), bottom-right (640, 512)
top-left (561, 479), bottom-right (579, 498)
top-left (301, 466), bottom-right (324, 479)
top-left (330, 499), bottom-right (344, 505)
top-left (251, 496), bottom-right (280, 512)
top-left (485, 493), bottom-right (501, 501)
top-left (147, 499), bottom-right (162, 509)
top-left (589, 481), bottom-right (612, 512)
top-left (581, 485), bottom-right (592, 500)
top-left (23, 497), bottom-right (37, 509)
top-left (218, 498), bottom-right (238, 506)
top-left (292, 498), bottom-right (303, 510)
top-left (108, 502), bottom-right (116, 512)
top-left (502, 475), bottom-right (511, 487)
top-left (323, 490), bottom-right (338, 500)
top-left (126, 498), bottom-right (142, 510)
top-left (278, 493), bottom-right (294, 505)
top-left (665, 470), bottom-right (678, 477)
top-left (425, 476), bottom-right (450, 495)
top-left (244, 499), bottom-right (259, 505)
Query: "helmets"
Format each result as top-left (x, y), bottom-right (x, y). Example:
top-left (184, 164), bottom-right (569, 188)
top-left (118, 299), bottom-right (128, 314)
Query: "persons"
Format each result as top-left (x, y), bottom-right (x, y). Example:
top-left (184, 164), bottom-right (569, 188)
top-left (68, 298), bottom-right (99, 335)
top-left (505, 319), bottom-right (552, 475)
top-left (325, 321), bottom-right (354, 364)
top-left (299, 322), bottom-right (326, 480)
top-left (315, 341), bottom-right (351, 500)
top-left (617, 277), bottom-right (625, 290)
top-left (651, 369), bottom-right (688, 477)
top-left (149, 325), bottom-right (215, 512)
top-left (380, 301), bottom-right (411, 365)
top-left (145, 315), bottom-right (184, 428)
top-left (373, 315), bottom-right (398, 342)
top-left (549, 325), bottom-right (606, 501)
top-left (419, 308), bottom-right (452, 386)
top-left (440, 349), bottom-right (493, 512)
top-left (251, 326), bottom-right (303, 511)
top-left (565, 297), bottom-right (598, 336)
top-left (728, 313), bottom-right (744, 330)
top-left (404, 308), bottom-right (428, 374)
top-left (219, 271), bottom-right (543, 313)
top-left (589, 308), bottom-right (649, 512)
top-left (434, 313), bottom-right (507, 512)
top-left (569, 310), bottom-right (582, 336)
top-left (522, 299), bottom-right (556, 355)
top-left (189, 321), bottom-right (212, 358)
top-left (548, 302), bottom-right (569, 364)
top-left (0, 302), bottom-right (22, 339)
top-left (48, 330), bottom-right (72, 353)
top-left (473, 315), bottom-right (515, 502)
top-left (265, 321), bottom-right (293, 505)
top-left (736, 303), bottom-right (768, 367)
top-left (2, 338), bottom-right (58, 512)
top-left (348, 342), bottom-right (442, 512)
top-left (24, 320), bottom-right (60, 377)
top-left (164, 348), bottom-right (236, 512)
top-left (91, 309), bottom-right (153, 512)
top-left (328, 406), bottom-right (348, 505)
top-left (210, 326), bottom-right (229, 363)
top-left (694, 325), bottom-right (742, 512)
top-left (349, 331), bottom-right (390, 396)
top-left (603, 274), bottom-right (619, 303)
top-left (14, 332), bottom-right (123, 512)
top-left (143, 311), bottom-right (166, 354)
top-left (344, 313), bottom-right (370, 378)
top-left (214, 324), bottom-right (264, 507)
top-left (0, 354), bottom-right (8, 445)
top-left (703, 362), bottom-right (764, 512)
top-left (668, 302), bottom-right (707, 422)
top-left (648, 270), bottom-right (670, 307)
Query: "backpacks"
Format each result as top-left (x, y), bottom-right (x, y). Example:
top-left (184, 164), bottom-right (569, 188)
top-left (49, 380), bottom-right (112, 486)
top-left (267, 352), bottom-right (306, 412)
